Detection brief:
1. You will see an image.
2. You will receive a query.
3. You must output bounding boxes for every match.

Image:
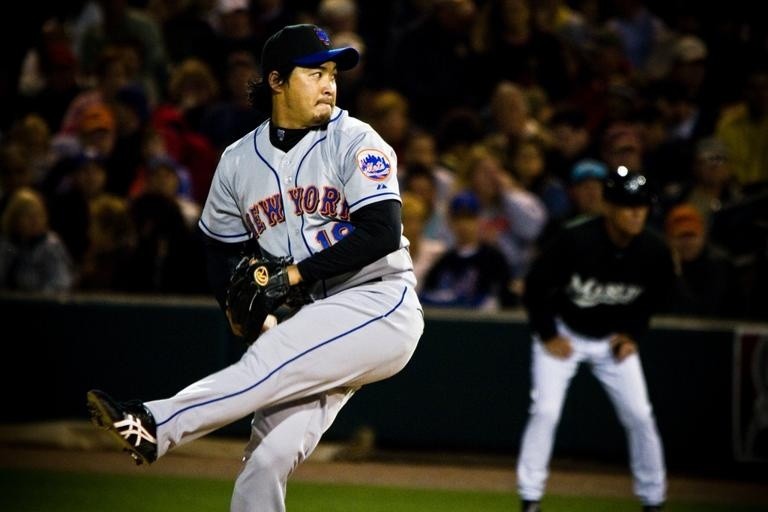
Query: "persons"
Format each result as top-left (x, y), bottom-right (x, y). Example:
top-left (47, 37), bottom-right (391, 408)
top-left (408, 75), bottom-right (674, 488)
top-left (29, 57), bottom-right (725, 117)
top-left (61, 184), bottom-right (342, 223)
top-left (87, 23), bottom-right (425, 512)
top-left (0, 0), bottom-right (768, 323)
top-left (517, 165), bottom-right (669, 512)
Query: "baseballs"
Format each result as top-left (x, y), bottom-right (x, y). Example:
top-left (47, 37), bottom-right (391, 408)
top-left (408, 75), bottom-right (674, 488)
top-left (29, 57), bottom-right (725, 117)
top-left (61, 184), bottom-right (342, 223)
top-left (260, 312), bottom-right (278, 333)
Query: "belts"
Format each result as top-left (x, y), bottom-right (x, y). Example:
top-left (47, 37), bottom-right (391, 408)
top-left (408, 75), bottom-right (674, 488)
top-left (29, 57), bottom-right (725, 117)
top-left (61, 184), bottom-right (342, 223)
top-left (326, 277), bottom-right (382, 298)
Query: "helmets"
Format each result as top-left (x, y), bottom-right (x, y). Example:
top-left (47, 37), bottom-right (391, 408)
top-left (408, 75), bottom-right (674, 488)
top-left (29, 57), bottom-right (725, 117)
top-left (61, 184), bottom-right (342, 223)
top-left (603, 168), bottom-right (656, 208)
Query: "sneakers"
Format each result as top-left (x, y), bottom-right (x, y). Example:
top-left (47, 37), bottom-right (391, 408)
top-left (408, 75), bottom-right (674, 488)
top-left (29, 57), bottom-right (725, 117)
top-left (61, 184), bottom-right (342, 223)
top-left (88, 389), bottom-right (157, 468)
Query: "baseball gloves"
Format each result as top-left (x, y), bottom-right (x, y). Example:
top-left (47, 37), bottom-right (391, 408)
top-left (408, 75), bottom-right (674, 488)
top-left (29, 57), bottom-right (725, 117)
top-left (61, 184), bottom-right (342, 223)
top-left (219, 249), bottom-right (296, 343)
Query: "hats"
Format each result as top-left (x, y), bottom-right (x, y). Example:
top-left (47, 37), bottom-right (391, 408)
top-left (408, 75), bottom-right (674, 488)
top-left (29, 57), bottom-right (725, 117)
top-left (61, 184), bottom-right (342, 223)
top-left (261, 24), bottom-right (357, 71)
